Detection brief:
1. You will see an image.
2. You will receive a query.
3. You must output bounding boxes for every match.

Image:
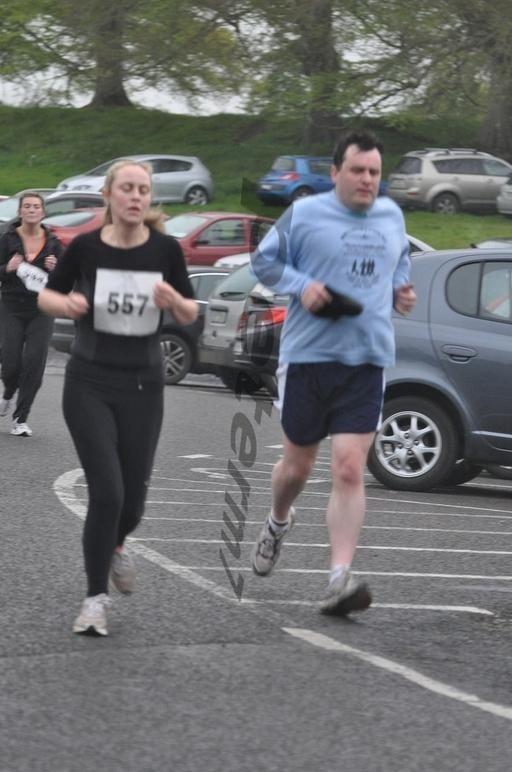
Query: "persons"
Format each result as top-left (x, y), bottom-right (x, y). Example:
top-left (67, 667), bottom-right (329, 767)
top-left (250, 133), bottom-right (416, 616)
top-left (1, 192), bottom-right (65, 437)
top-left (37, 159), bottom-right (199, 637)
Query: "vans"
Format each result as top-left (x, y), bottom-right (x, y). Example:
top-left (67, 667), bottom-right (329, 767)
top-left (56, 153), bottom-right (216, 206)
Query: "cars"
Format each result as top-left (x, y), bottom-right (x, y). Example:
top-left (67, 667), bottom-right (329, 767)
top-left (211, 234), bottom-right (436, 269)
top-left (371, 236), bottom-right (511, 491)
top-left (260, 154), bottom-right (387, 206)
top-left (498, 174), bottom-right (512, 216)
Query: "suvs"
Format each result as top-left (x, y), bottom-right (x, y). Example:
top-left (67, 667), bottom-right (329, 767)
top-left (386, 147), bottom-right (511, 215)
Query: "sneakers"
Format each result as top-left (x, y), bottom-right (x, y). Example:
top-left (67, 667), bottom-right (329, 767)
top-left (319, 572), bottom-right (372, 615)
top-left (10, 421), bottom-right (31, 436)
top-left (252, 505), bottom-right (295, 576)
top-left (109, 550), bottom-right (136, 595)
top-left (74, 594), bottom-right (111, 636)
top-left (0, 393), bottom-right (16, 416)
